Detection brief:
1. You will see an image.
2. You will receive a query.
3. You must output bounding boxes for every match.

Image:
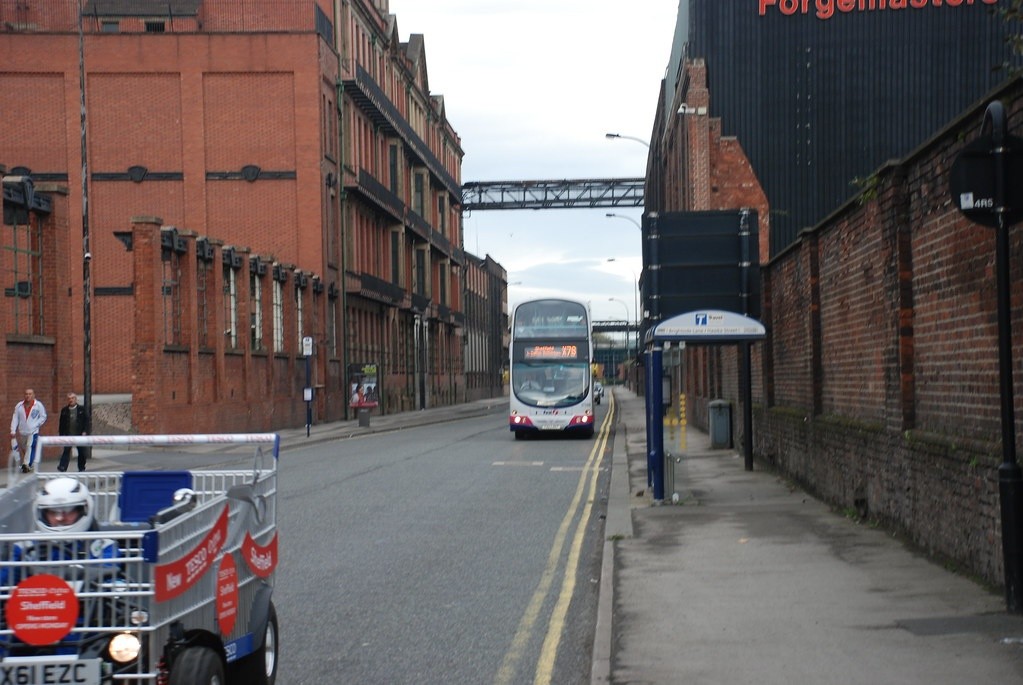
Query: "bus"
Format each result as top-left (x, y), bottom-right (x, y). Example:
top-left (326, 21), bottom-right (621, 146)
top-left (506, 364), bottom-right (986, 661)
top-left (502, 297), bottom-right (599, 436)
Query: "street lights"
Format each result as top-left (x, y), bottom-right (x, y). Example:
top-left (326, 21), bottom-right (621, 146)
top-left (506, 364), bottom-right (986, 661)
top-left (608, 258), bottom-right (640, 396)
top-left (608, 298), bottom-right (632, 391)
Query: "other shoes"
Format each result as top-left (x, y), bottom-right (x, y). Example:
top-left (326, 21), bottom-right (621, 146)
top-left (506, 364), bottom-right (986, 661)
top-left (22, 464), bottom-right (28, 473)
top-left (57, 465), bottom-right (65, 471)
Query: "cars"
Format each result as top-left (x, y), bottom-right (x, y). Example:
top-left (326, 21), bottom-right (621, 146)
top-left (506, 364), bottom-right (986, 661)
top-left (594, 381), bottom-right (604, 405)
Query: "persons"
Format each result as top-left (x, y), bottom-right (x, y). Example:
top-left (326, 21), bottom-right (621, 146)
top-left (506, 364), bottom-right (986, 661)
top-left (521, 373), bottom-right (541, 391)
top-left (57, 392), bottom-right (88, 472)
top-left (352, 384), bottom-right (376, 402)
top-left (10, 389), bottom-right (47, 473)
top-left (0, 478), bottom-right (133, 604)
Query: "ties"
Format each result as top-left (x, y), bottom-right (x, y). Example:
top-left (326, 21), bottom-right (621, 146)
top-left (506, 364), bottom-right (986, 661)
top-left (529, 381), bottom-right (531, 389)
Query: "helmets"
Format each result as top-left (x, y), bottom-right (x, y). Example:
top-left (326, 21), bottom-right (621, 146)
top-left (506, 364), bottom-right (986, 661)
top-left (32, 477), bottom-right (95, 543)
